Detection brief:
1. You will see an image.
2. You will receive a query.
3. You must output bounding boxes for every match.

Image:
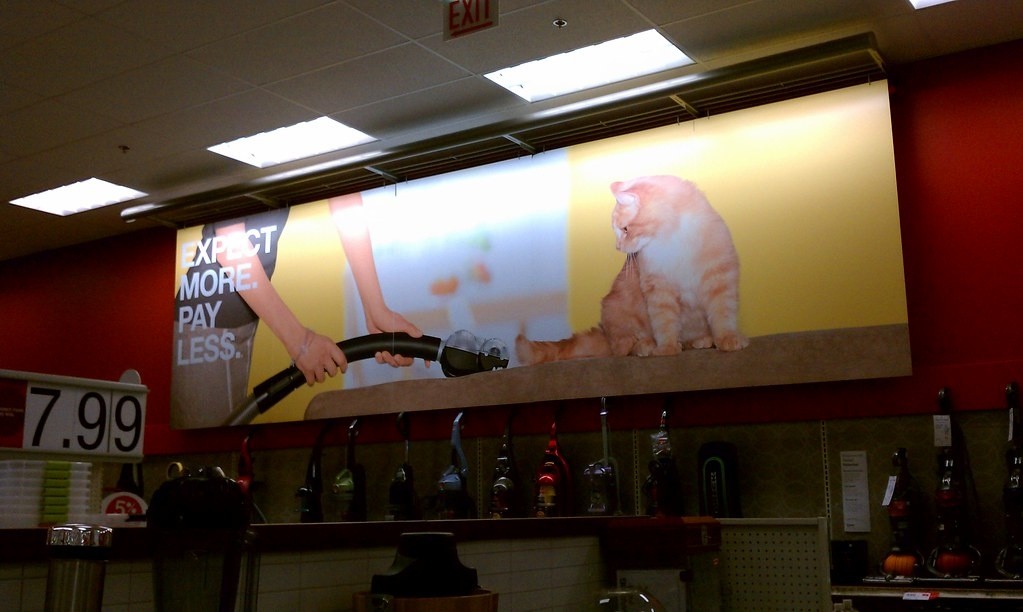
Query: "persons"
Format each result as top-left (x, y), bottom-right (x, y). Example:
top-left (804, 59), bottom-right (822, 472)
top-left (172, 191), bottom-right (430, 430)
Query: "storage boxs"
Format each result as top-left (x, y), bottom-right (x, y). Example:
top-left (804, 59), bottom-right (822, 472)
top-left (0, 461), bottom-right (92, 528)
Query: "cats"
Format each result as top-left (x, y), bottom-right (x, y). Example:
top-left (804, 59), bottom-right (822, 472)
top-left (515, 175), bottom-right (751, 367)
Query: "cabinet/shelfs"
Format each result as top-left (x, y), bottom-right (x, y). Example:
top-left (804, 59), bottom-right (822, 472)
top-left (830, 579), bottom-right (1023, 612)
